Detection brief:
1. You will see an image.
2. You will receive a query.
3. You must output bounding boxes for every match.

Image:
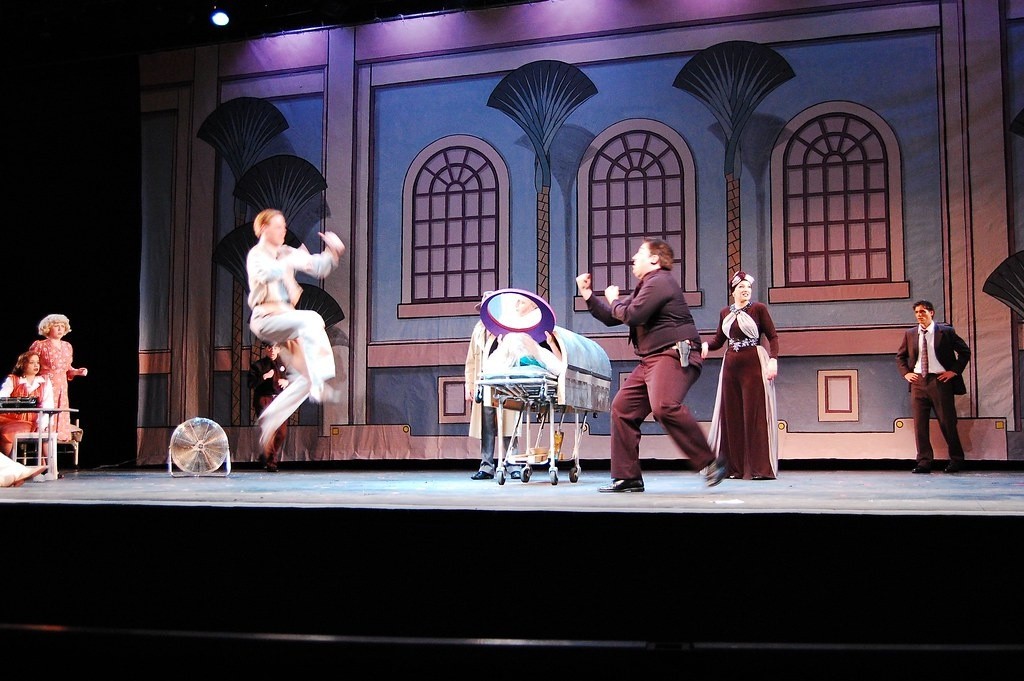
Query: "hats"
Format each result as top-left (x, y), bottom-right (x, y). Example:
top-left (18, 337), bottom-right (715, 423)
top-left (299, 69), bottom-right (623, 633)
top-left (730, 270), bottom-right (755, 291)
top-left (473, 291), bottom-right (494, 310)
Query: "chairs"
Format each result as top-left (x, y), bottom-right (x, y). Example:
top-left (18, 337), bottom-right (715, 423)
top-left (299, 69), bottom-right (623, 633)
top-left (11, 433), bottom-right (58, 482)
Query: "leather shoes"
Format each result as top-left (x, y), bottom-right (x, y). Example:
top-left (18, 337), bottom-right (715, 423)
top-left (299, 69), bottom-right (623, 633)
top-left (706, 459), bottom-right (729, 487)
top-left (599, 477), bottom-right (645, 493)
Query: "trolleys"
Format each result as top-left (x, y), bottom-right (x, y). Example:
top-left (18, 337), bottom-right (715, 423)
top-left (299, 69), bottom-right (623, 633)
top-left (476, 365), bottom-right (598, 486)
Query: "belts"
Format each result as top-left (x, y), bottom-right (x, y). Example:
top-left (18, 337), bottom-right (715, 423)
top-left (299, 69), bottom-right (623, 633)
top-left (689, 344), bottom-right (703, 352)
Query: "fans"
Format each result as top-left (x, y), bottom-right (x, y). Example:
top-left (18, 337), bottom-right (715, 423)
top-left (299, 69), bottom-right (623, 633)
top-left (169, 418), bottom-right (231, 476)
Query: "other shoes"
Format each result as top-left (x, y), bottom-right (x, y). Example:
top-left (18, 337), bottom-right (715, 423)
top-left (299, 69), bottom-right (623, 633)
top-left (267, 467), bottom-right (279, 471)
top-left (912, 465), bottom-right (931, 473)
top-left (511, 472), bottom-right (521, 481)
top-left (470, 471), bottom-right (494, 480)
top-left (944, 466), bottom-right (960, 473)
top-left (57, 473), bottom-right (62, 478)
top-left (12, 464), bottom-right (48, 489)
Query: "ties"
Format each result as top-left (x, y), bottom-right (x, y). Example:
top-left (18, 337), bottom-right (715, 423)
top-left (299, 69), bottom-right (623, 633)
top-left (921, 329), bottom-right (929, 379)
top-left (628, 278), bottom-right (644, 346)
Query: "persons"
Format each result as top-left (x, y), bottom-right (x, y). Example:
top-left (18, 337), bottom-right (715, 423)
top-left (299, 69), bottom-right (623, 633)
top-left (465, 291), bottom-right (523, 479)
top-left (897, 301), bottom-right (971, 473)
top-left (249, 340), bottom-right (291, 471)
top-left (29, 315), bottom-right (87, 477)
top-left (246, 210), bottom-right (346, 449)
top-left (576, 238), bottom-right (726, 493)
top-left (0, 352), bottom-right (53, 458)
top-left (701, 272), bottom-right (779, 480)
top-left (484, 332), bottom-right (561, 375)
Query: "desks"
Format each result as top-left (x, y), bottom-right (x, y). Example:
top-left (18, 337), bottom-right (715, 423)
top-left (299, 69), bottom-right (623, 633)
top-left (0, 409), bottom-right (79, 481)
